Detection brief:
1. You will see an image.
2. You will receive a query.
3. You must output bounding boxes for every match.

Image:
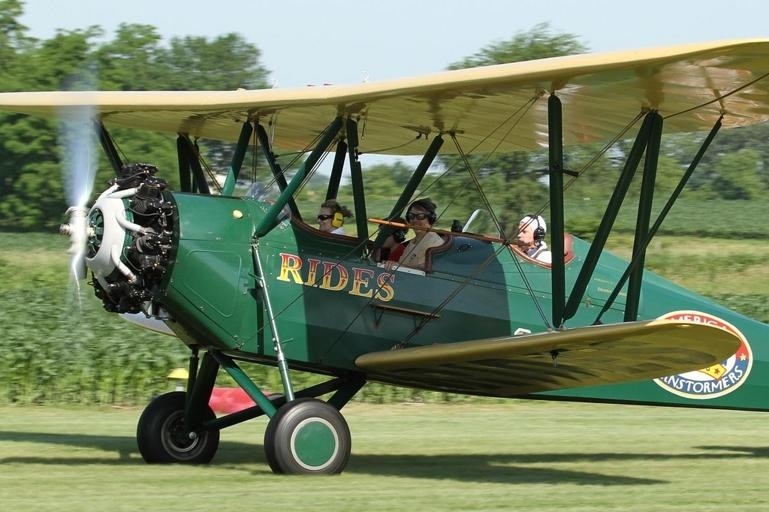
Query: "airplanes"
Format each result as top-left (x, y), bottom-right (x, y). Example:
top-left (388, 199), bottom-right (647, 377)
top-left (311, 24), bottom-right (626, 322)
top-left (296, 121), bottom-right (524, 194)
top-left (1, 33), bottom-right (769, 474)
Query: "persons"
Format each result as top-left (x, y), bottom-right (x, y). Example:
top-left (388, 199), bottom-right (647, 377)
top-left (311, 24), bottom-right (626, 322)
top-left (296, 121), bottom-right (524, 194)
top-left (378, 198), bottom-right (447, 273)
top-left (513, 214), bottom-right (552, 264)
top-left (374, 216), bottom-right (410, 263)
top-left (316, 198), bottom-right (356, 237)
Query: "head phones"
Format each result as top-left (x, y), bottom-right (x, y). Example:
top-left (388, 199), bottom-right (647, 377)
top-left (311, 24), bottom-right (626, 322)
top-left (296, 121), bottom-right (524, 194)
top-left (406, 200), bottom-right (437, 225)
top-left (524, 216), bottom-right (546, 243)
top-left (395, 227), bottom-right (406, 243)
top-left (331, 212), bottom-right (344, 228)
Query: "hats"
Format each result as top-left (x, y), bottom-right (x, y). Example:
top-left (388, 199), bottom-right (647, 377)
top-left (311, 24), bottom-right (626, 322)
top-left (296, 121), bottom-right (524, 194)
top-left (520, 214), bottom-right (547, 235)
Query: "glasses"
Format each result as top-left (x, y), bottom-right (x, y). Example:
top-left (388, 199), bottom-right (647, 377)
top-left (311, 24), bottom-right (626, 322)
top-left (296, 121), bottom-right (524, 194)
top-left (317, 214), bottom-right (333, 220)
top-left (406, 212), bottom-right (427, 220)
